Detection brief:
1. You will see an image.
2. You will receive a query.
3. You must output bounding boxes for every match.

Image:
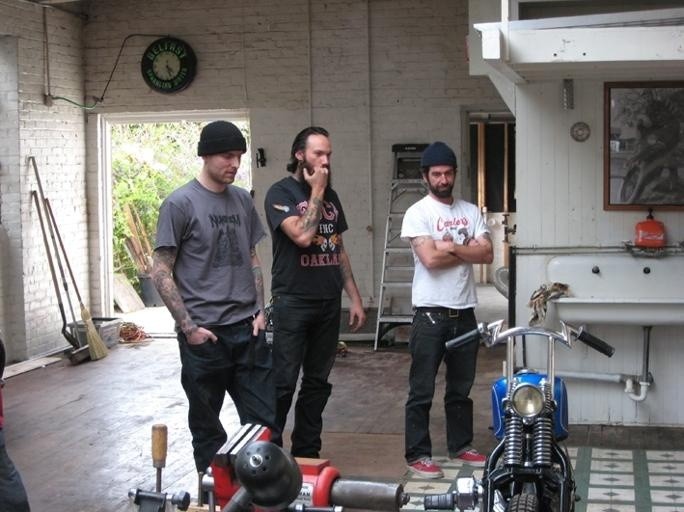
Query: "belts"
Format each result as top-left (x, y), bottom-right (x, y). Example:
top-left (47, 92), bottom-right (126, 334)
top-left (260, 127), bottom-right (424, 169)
top-left (416, 308), bottom-right (466, 318)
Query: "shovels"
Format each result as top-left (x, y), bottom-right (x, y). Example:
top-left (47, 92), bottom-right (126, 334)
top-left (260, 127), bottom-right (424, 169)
top-left (31, 191), bottom-right (78, 350)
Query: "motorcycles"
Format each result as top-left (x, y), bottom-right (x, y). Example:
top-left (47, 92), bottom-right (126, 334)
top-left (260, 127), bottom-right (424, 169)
top-left (442, 317), bottom-right (616, 511)
top-left (617, 120), bottom-right (665, 205)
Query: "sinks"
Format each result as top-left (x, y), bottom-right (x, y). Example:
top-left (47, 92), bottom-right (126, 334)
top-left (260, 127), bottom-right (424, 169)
top-left (545, 253), bottom-right (684, 327)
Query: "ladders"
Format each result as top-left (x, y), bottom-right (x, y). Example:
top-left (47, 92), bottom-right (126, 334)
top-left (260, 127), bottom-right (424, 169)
top-left (374, 144), bottom-right (429, 355)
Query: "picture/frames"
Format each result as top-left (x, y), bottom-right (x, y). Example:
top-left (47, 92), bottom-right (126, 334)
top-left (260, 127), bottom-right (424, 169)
top-left (603, 80), bottom-right (684, 211)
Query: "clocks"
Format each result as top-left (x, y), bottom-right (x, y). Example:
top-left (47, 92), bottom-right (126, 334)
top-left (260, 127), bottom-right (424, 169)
top-left (141, 37), bottom-right (197, 94)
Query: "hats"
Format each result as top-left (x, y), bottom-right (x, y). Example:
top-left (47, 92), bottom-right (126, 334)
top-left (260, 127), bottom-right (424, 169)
top-left (197, 121), bottom-right (246, 156)
top-left (420, 141), bottom-right (457, 168)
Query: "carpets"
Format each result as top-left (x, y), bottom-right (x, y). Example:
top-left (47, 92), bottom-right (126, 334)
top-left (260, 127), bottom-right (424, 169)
top-left (335, 446), bottom-right (684, 512)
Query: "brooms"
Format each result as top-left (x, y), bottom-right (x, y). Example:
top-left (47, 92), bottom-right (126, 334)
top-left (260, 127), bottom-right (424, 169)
top-left (44, 197), bottom-right (109, 362)
top-left (30, 156), bottom-right (90, 366)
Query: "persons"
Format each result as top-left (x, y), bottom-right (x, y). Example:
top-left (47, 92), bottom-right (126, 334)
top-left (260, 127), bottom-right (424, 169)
top-left (636, 91), bottom-right (674, 128)
top-left (149, 119), bottom-right (283, 474)
top-left (398, 141), bottom-right (494, 478)
top-left (262, 127), bottom-right (366, 458)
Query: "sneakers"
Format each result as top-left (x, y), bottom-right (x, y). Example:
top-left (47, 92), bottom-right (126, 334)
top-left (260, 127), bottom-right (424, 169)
top-left (407, 456), bottom-right (443, 480)
top-left (448, 448), bottom-right (486, 467)
